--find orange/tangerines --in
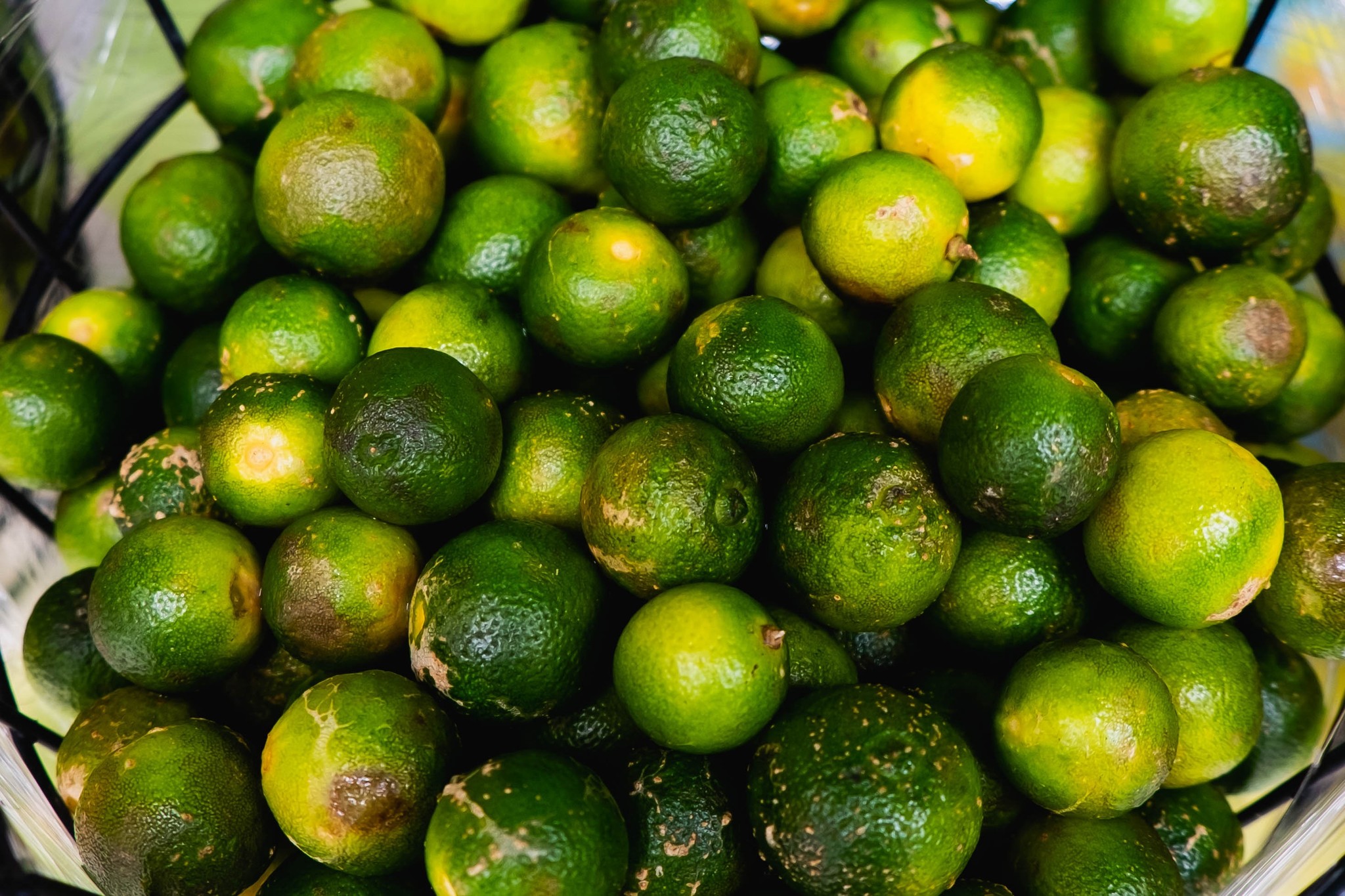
[0,0,1345,896]
